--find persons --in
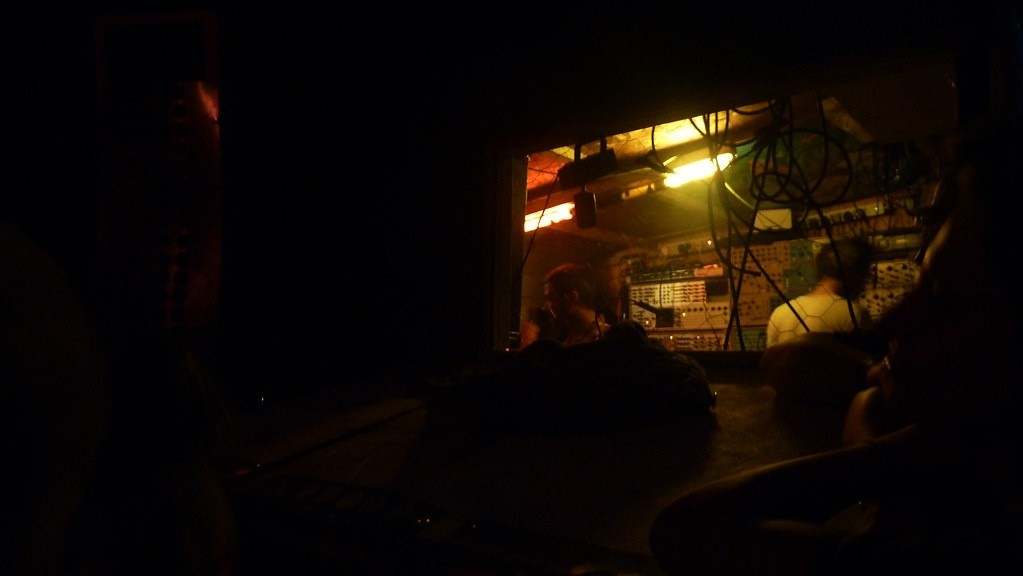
[541,262,609,345]
[764,239,873,348]
[0,221,113,576]
